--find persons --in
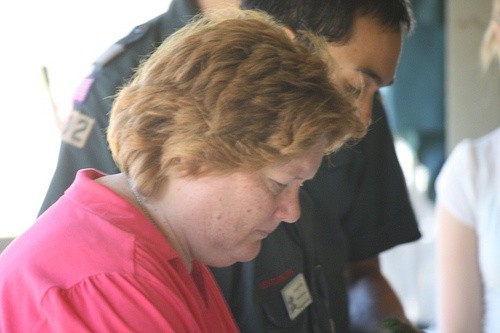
[0,3,368,332]
[435,0,500,332]
[205,0,416,333]
[37,0,423,333]
